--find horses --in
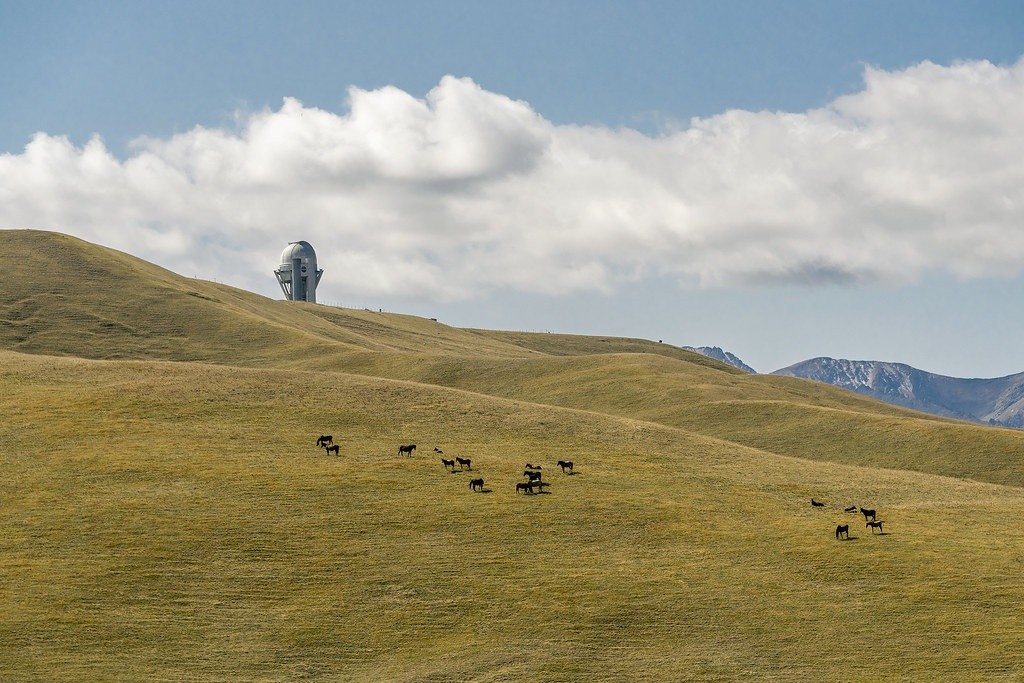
[528,475,543,493]
[321,443,340,456]
[515,482,529,494]
[865,521,883,534]
[441,458,455,470]
[859,507,877,521]
[844,506,857,513]
[470,478,484,491]
[433,447,443,454]
[811,498,824,506]
[316,434,333,446]
[398,444,416,457]
[456,457,471,469]
[525,463,542,469]
[523,470,542,481]
[556,460,573,473]
[835,524,849,540]
